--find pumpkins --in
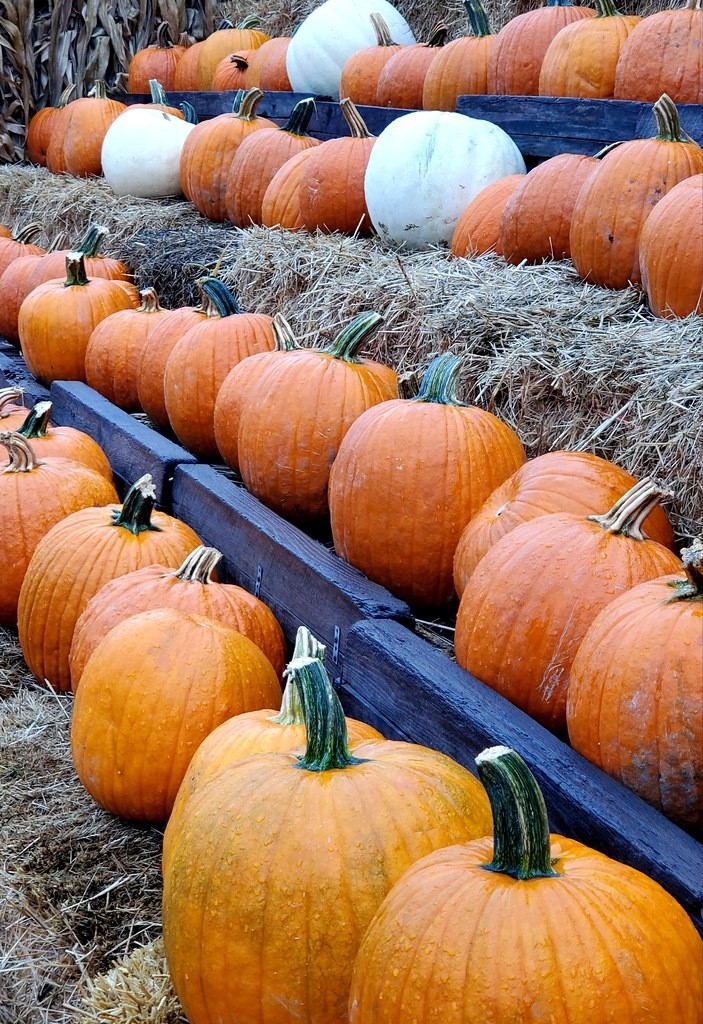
[0,1,700,1024]
